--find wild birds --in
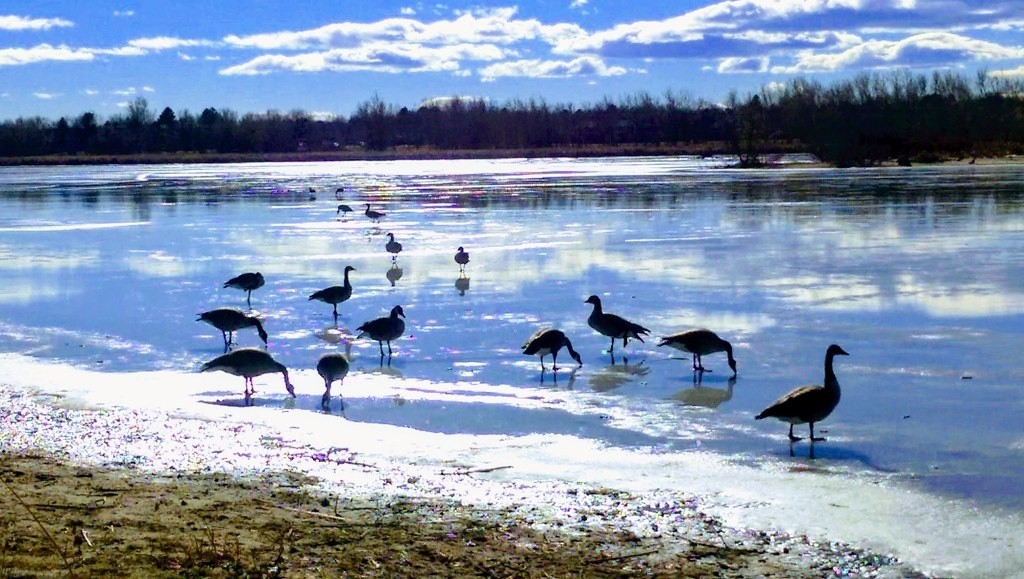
[308,265,357,311]
[335,188,344,194]
[336,205,353,215]
[521,327,582,370]
[364,203,386,223]
[753,344,849,441]
[657,328,736,374]
[583,295,651,352]
[195,309,267,344]
[317,353,349,407]
[385,233,402,257]
[356,305,406,357]
[454,247,470,269]
[309,187,316,193]
[200,349,296,398]
[223,272,265,301]
